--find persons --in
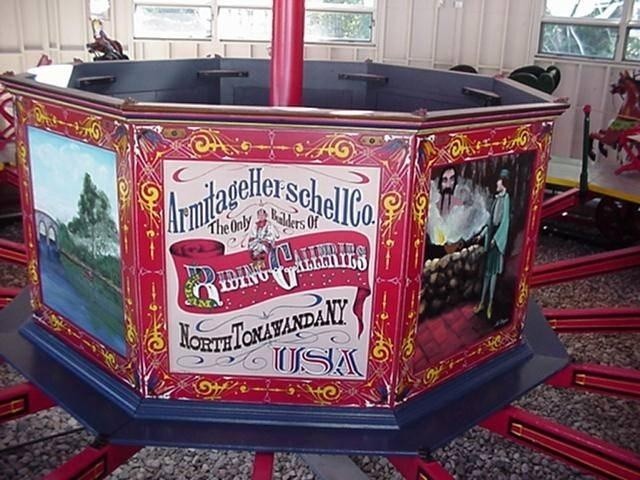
[436,165,464,219]
[249,208,280,260]
[472,169,513,320]
